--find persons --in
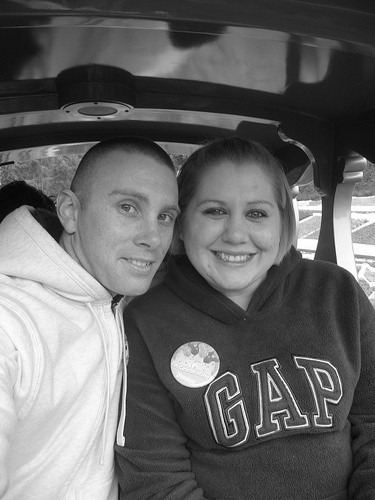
[113,135,375,500]
[0,136,181,500]
